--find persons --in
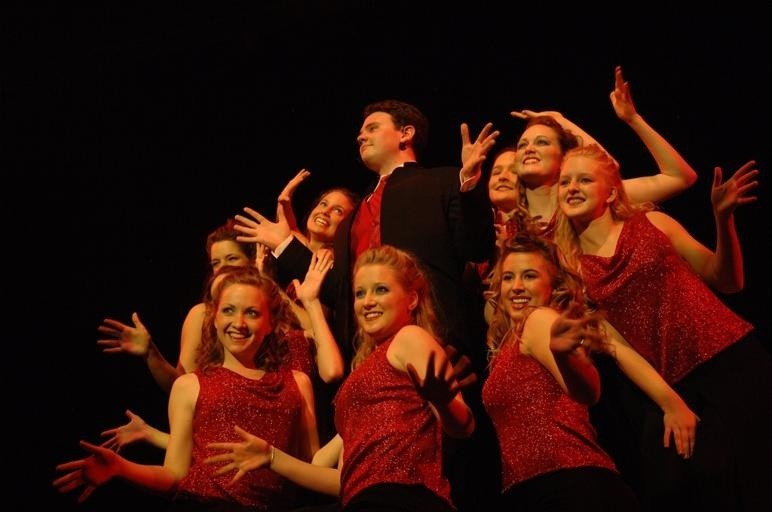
[49,61,772,511]
